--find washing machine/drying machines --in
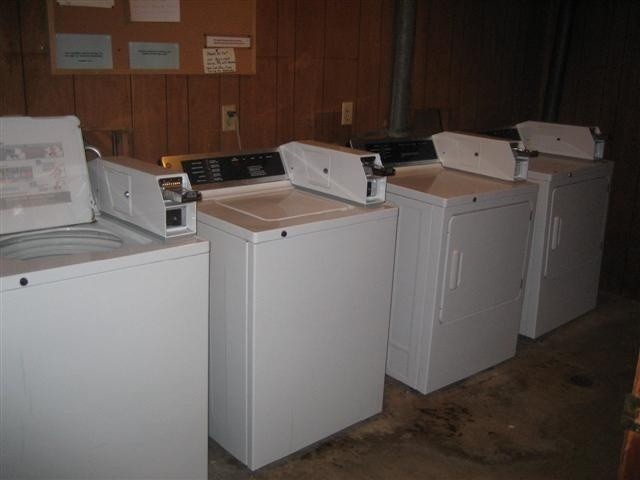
[342,129,542,397]
[463,118,616,341]
[1,114,214,480]
[158,139,401,471]
